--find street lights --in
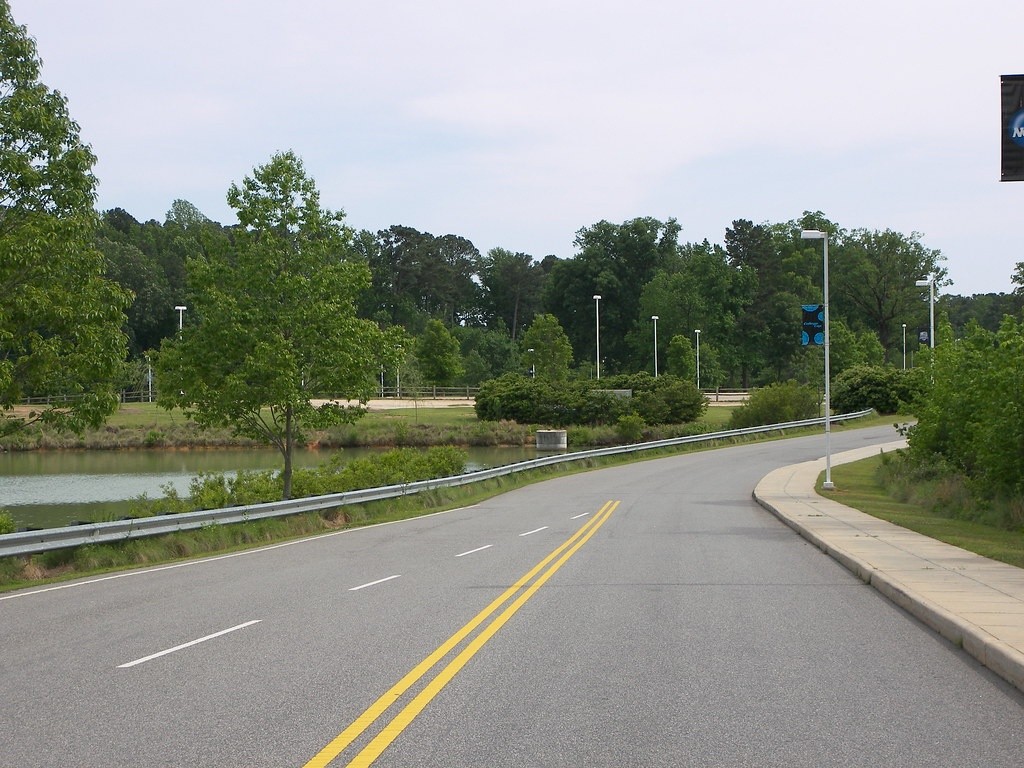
[145,355,152,402]
[652,315,668,376]
[394,344,402,397]
[695,329,702,390]
[800,230,836,490]
[175,306,187,394]
[593,295,601,381]
[902,324,907,367]
[916,272,936,383]
